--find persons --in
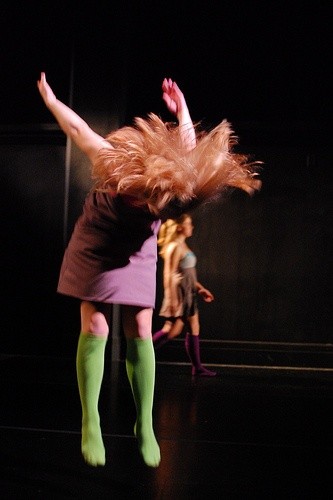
[35,71,265,469]
[152,212,218,377]
